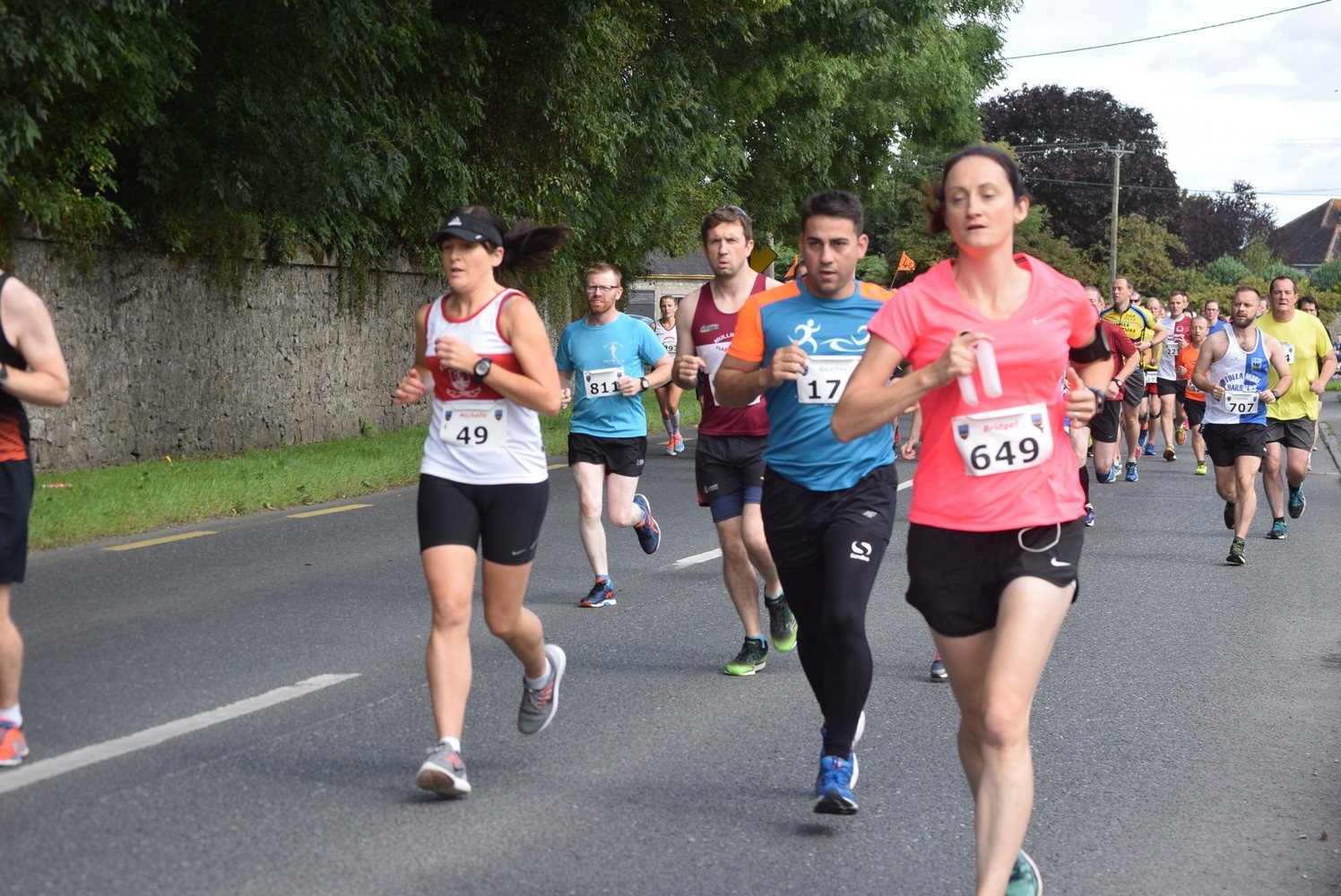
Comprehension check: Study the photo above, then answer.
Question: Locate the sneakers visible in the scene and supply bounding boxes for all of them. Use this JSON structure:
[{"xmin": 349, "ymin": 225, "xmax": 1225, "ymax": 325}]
[
  {"xmin": 724, "ymin": 637, "xmax": 769, "ymax": 676},
  {"xmin": 580, "ymin": 581, "xmax": 617, "ymax": 607},
  {"xmin": 517, "ymin": 643, "xmax": 567, "ymax": 735},
  {"xmin": 820, "ymin": 710, "xmax": 865, "ymax": 750},
  {"xmin": 1085, "ymin": 507, "xmax": 1095, "ymax": 526},
  {"xmin": 1195, "ymin": 462, "xmax": 1207, "ymax": 475},
  {"xmin": 764, "ymin": 582, "xmax": 798, "ymax": 653},
  {"xmin": 1223, "ymin": 501, "xmax": 1235, "ymax": 529},
  {"xmin": 1267, "ymin": 521, "xmax": 1289, "ymax": 539},
  {"xmin": 930, "ymin": 651, "xmax": 948, "ymax": 678},
  {"xmin": 632, "ymin": 494, "xmax": 661, "ymax": 555},
  {"xmin": 415, "ymin": 742, "xmax": 472, "ymax": 798},
  {"xmin": 812, "ymin": 749, "xmax": 860, "ymax": 815},
  {"xmin": 665, "ymin": 438, "xmax": 677, "ymax": 456},
  {"xmin": 674, "ymin": 434, "xmax": 685, "ymax": 452},
  {"xmin": 0, "ymin": 717, "xmax": 30, "ymax": 766},
  {"xmin": 1005, "ymin": 848, "xmax": 1043, "ymax": 896},
  {"xmin": 1225, "ymin": 539, "xmax": 1246, "ymax": 564},
  {"xmin": 1110, "ymin": 463, "xmax": 1122, "ymax": 481},
  {"xmin": 1125, "ymin": 462, "xmax": 1138, "ymax": 482},
  {"xmin": 1117, "ymin": 418, "xmax": 1190, "ymax": 462},
  {"xmin": 1287, "ymin": 480, "xmax": 1306, "ymax": 519}
]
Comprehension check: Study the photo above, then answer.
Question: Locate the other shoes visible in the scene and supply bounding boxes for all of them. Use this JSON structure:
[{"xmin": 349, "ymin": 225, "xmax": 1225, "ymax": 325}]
[{"xmin": 894, "ymin": 430, "xmax": 901, "ymax": 442}]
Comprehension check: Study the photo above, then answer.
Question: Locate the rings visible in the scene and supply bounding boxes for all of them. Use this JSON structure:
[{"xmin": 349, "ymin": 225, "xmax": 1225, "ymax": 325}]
[{"xmin": 1075, "ymin": 412, "xmax": 1079, "ymax": 419}]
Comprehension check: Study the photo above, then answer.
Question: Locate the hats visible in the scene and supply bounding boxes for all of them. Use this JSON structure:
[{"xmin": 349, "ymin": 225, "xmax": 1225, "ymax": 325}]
[{"xmin": 427, "ymin": 212, "xmax": 502, "ymax": 247}]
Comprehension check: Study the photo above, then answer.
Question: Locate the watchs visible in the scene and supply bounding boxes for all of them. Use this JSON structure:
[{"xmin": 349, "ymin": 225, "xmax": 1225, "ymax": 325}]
[
  {"xmin": 639, "ymin": 377, "xmax": 649, "ymax": 392},
  {"xmin": 472, "ymin": 356, "xmax": 492, "ymax": 384},
  {"xmin": 1150, "ymin": 341, "xmax": 1154, "ymax": 348},
  {"xmin": 1088, "ymin": 387, "xmax": 1105, "ymax": 414},
  {"xmin": 1111, "ymin": 377, "xmax": 1123, "ymax": 388},
  {"xmin": 1271, "ymin": 390, "xmax": 1279, "ymax": 401}
]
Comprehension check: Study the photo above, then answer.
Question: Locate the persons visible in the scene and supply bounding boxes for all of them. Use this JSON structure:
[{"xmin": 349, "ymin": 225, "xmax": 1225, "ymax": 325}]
[
  {"xmin": 555, "ymin": 262, "xmax": 674, "ymax": 609},
  {"xmin": 712, "ymin": 191, "xmax": 1338, "ymax": 812},
  {"xmin": 392, "ymin": 207, "xmax": 575, "ymax": 798},
  {"xmin": 649, "ymin": 296, "xmax": 684, "ymax": 456},
  {"xmin": 829, "ymin": 142, "xmax": 1113, "ymax": 896},
  {"xmin": 669, "ymin": 206, "xmax": 798, "ymax": 676},
  {"xmin": 1, "ymin": 268, "xmax": 70, "ymax": 767}
]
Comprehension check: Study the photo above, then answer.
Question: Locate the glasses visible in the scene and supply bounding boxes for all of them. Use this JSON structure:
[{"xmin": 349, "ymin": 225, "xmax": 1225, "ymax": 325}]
[
  {"xmin": 586, "ymin": 284, "xmax": 620, "ymax": 294},
  {"xmin": 713, "ymin": 206, "xmax": 748, "ymax": 219}
]
